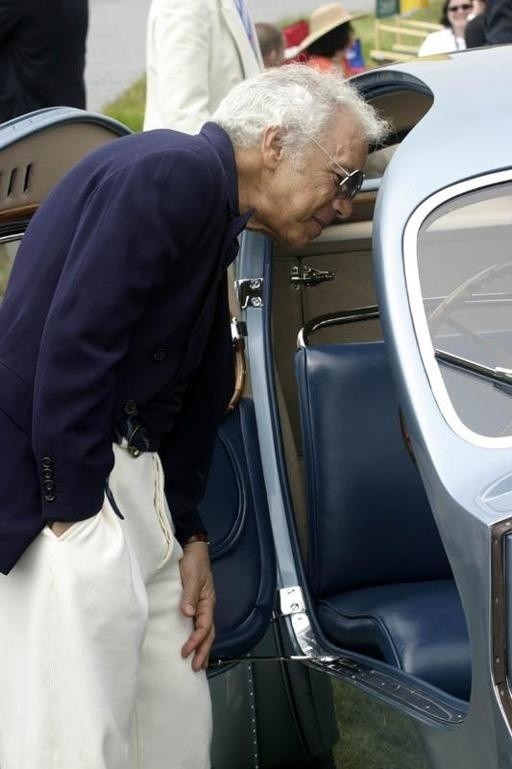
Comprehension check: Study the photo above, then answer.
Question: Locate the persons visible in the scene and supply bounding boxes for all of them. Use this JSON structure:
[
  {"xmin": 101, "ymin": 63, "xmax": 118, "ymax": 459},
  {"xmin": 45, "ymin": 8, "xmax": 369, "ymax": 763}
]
[
  {"xmin": 255, "ymin": 20, "xmax": 284, "ymax": 68},
  {"xmin": 142, "ymin": 0, "xmax": 265, "ymax": 133},
  {"xmin": 296, "ymin": 4, "xmax": 370, "ymax": 79},
  {"xmin": 1, "ymin": 55, "xmax": 390, "ymax": 768},
  {"xmin": 419, "ymin": 0, "xmax": 478, "ymax": 58},
  {"xmin": 346, "ymin": 29, "xmax": 367, "ymax": 74},
  {"xmin": 0, "ymin": 0, "xmax": 88, "ymax": 119},
  {"xmin": 465, "ymin": 0, "xmax": 512, "ymax": 49}
]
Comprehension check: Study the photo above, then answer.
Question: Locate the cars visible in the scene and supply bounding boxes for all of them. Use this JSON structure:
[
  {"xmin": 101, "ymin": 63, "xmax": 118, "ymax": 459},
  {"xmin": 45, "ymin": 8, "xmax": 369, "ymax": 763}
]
[{"xmin": 1, "ymin": 45, "xmax": 512, "ymax": 769}]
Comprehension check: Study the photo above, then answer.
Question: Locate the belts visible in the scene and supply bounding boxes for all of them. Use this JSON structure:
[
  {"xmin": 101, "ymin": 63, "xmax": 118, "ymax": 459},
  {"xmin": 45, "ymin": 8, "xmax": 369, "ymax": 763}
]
[{"xmin": 120, "ymin": 418, "xmax": 161, "ymax": 458}]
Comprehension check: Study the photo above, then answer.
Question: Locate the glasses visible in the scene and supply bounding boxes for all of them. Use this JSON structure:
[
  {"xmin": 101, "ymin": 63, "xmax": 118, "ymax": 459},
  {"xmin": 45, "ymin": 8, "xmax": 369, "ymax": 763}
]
[
  {"xmin": 448, "ymin": 5, "xmax": 472, "ymax": 11},
  {"xmin": 310, "ymin": 137, "xmax": 363, "ymax": 200}
]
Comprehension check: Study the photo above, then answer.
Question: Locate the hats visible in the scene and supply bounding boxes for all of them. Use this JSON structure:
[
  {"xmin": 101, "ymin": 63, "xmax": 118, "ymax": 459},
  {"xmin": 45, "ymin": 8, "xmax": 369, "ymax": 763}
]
[{"xmin": 298, "ymin": 4, "xmax": 369, "ymax": 52}]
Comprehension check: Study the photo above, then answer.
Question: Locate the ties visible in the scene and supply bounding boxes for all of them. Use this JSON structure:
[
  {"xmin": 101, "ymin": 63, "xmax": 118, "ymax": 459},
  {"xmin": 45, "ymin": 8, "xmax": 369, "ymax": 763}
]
[{"xmin": 234, "ymin": 0, "xmax": 252, "ymax": 41}]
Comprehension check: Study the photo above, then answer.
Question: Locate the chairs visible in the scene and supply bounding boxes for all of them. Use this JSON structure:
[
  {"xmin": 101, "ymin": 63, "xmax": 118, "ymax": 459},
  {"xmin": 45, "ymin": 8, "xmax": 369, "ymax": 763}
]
[{"xmin": 289, "ymin": 329, "xmax": 511, "ymax": 698}]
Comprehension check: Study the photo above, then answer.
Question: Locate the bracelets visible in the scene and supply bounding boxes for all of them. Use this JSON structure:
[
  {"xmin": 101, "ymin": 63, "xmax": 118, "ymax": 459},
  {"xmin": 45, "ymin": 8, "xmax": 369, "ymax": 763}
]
[{"xmin": 186, "ymin": 534, "xmax": 209, "ymax": 545}]
